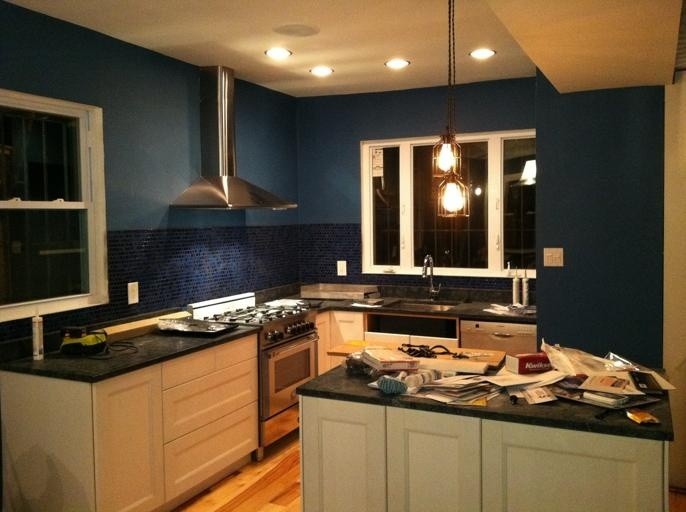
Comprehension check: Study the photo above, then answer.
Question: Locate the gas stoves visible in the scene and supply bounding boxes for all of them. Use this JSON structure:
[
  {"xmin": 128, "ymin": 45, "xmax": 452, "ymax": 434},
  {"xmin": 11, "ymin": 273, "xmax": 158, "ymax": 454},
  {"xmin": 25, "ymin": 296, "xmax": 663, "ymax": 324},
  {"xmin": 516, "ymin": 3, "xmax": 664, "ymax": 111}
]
[{"xmin": 202, "ymin": 302, "xmax": 317, "ymax": 346}]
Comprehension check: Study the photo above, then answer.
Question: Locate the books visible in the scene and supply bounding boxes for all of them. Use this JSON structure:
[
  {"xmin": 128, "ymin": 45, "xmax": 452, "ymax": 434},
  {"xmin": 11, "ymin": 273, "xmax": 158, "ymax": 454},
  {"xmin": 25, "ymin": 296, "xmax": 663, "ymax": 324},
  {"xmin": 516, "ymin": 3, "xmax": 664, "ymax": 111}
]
[{"xmin": 363, "ymin": 349, "xmax": 678, "ymax": 410}]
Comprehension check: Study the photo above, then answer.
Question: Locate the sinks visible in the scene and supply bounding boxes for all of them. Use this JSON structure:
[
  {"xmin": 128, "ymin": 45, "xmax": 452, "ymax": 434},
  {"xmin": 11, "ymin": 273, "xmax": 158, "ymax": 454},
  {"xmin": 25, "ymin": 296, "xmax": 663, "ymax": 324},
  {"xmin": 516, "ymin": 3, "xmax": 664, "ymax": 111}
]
[{"xmin": 383, "ymin": 299, "xmax": 461, "ymax": 312}]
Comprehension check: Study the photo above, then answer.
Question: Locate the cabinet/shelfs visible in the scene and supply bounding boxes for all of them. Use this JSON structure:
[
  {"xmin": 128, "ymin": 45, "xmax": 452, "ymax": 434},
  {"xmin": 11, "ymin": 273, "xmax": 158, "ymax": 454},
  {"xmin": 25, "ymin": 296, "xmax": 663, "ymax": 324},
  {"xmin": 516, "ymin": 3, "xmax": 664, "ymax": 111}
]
[
  {"xmin": 302, "ymin": 396, "xmax": 481, "ymax": 512},
  {"xmin": 315, "ymin": 311, "xmax": 366, "ymax": 374},
  {"xmin": 162, "ymin": 333, "xmax": 259, "ymax": 503},
  {"xmin": 92, "ymin": 363, "xmax": 165, "ymax": 512},
  {"xmin": 481, "ymin": 419, "xmax": 664, "ymax": 512},
  {"xmin": 364, "ymin": 332, "xmax": 458, "ymax": 348}
]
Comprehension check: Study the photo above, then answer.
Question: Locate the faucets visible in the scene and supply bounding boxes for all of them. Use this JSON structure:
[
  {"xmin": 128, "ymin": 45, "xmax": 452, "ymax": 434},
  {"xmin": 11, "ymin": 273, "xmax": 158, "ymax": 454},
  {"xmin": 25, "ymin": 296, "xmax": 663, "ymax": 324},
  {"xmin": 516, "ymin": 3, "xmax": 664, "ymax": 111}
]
[{"xmin": 422, "ymin": 255, "xmax": 441, "ymax": 301}]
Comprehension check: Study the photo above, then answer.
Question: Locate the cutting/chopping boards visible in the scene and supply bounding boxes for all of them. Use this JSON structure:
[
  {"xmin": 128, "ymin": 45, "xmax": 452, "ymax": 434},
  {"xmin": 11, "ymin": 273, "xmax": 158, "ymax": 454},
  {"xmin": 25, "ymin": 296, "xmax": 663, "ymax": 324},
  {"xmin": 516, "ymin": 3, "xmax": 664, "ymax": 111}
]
[{"xmin": 327, "ymin": 340, "xmax": 506, "ymax": 370}]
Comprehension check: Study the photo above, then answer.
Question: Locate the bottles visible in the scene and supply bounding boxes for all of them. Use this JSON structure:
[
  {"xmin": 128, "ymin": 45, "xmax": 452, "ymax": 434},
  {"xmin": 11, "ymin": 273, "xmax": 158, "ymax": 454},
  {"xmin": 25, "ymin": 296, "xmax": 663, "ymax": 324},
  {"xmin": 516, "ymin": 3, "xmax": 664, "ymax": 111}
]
[
  {"xmin": 521, "ymin": 269, "xmax": 530, "ymax": 306},
  {"xmin": 31, "ymin": 309, "xmax": 45, "ymax": 361},
  {"xmin": 512, "ymin": 269, "xmax": 521, "ymax": 307}
]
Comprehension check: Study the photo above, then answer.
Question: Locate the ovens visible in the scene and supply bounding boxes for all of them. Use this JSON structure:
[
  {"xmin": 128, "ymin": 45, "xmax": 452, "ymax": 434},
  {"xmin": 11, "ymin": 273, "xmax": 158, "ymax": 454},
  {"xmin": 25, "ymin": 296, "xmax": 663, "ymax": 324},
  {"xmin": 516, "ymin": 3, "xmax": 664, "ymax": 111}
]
[{"xmin": 259, "ymin": 331, "xmax": 319, "ymax": 448}]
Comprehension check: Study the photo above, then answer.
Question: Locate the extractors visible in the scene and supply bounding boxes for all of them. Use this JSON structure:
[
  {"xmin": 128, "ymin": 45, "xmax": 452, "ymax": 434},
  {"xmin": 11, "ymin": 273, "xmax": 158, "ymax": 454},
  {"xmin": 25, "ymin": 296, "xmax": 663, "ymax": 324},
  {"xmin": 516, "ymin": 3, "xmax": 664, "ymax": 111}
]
[{"xmin": 169, "ymin": 65, "xmax": 299, "ymax": 211}]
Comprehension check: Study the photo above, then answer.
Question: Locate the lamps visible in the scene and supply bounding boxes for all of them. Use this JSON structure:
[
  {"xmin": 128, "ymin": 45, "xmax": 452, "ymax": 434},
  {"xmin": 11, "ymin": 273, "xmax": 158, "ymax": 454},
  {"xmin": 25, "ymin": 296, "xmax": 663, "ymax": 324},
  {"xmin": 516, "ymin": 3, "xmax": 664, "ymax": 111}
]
[
  {"xmin": 437, "ymin": 0, "xmax": 471, "ymax": 218},
  {"xmin": 432, "ymin": 0, "xmax": 462, "ymax": 178}
]
